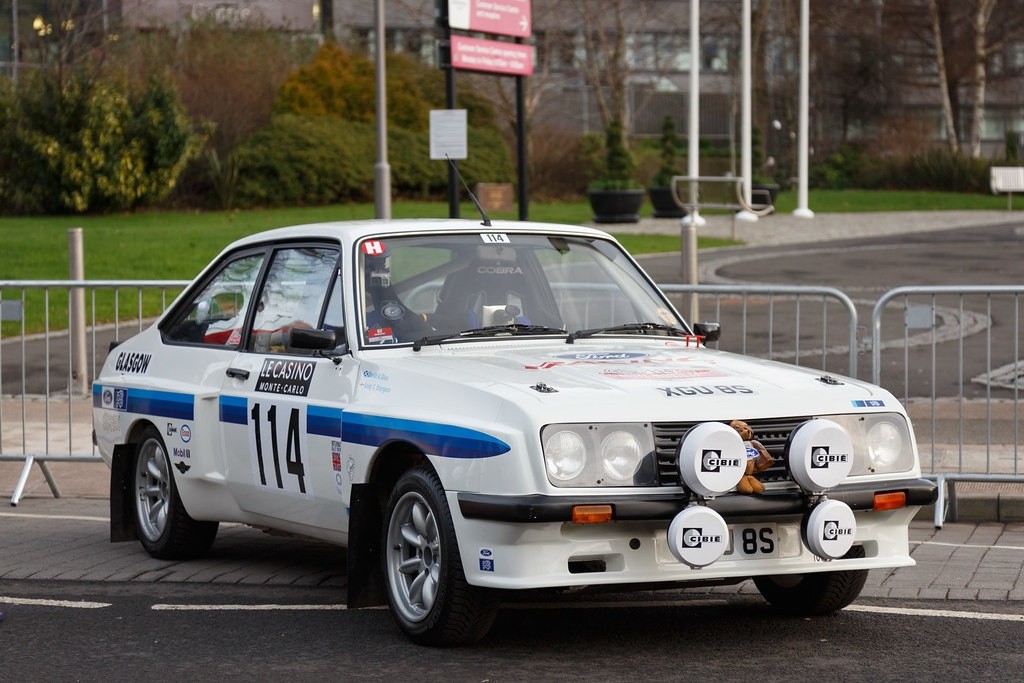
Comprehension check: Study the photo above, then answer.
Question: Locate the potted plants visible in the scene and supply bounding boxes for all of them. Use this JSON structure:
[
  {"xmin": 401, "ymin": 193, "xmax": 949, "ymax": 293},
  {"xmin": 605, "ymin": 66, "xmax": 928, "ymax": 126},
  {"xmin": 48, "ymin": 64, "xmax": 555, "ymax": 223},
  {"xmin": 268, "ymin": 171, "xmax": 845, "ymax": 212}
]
[
  {"xmin": 749, "ymin": 126, "xmax": 777, "ymax": 215},
  {"xmin": 589, "ymin": 114, "xmax": 646, "ymax": 224},
  {"xmin": 649, "ymin": 113, "xmax": 688, "ymax": 217}
]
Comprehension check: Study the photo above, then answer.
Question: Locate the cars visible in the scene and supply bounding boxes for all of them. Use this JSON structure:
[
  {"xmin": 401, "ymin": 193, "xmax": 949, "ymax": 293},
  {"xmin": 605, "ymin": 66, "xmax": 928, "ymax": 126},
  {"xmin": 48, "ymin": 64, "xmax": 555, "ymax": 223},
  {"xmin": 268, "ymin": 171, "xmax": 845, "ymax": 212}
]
[{"xmin": 92, "ymin": 219, "xmax": 940, "ymax": 648}]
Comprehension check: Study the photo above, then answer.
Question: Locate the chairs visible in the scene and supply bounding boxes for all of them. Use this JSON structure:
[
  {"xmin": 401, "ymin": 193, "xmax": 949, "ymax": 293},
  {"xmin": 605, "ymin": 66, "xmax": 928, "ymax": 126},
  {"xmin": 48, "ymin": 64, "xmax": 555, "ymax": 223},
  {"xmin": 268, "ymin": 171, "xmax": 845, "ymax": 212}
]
[{"xmin": 432, "ymin": 259, "xmax": 560, "ymax": 332}]
